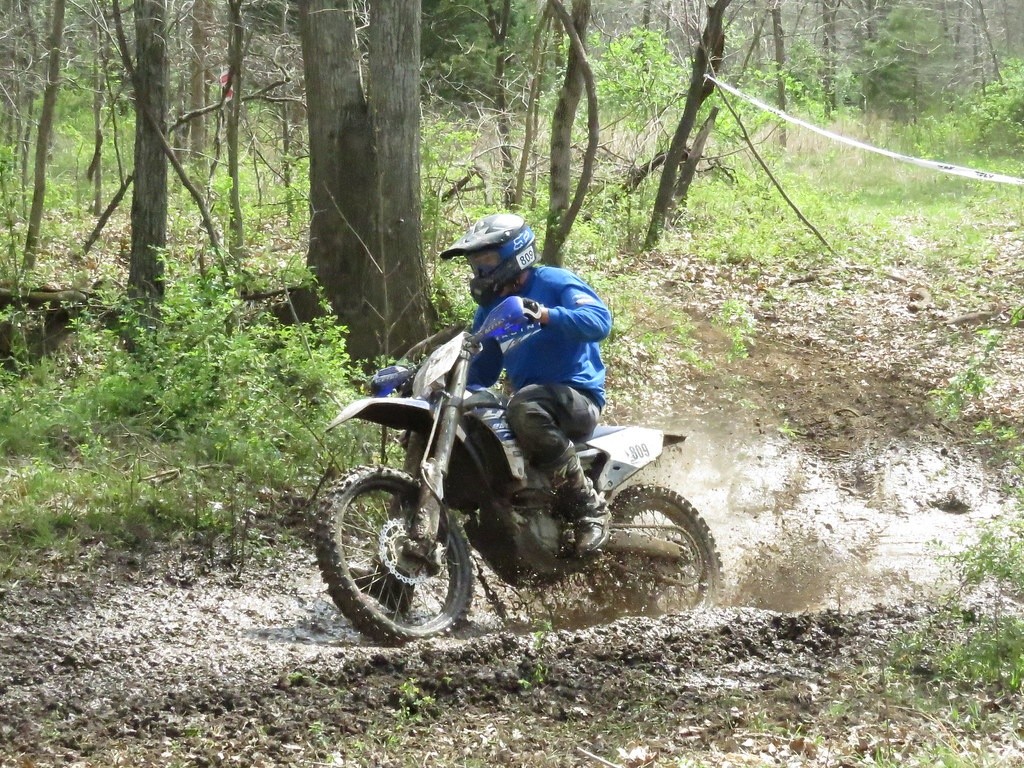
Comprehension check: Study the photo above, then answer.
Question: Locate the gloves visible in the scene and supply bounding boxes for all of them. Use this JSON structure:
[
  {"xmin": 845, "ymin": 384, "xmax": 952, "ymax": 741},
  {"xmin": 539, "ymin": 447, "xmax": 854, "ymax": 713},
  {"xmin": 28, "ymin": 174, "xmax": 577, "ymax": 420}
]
[{"xmin": 522, "ymin": 298, "xmax": 540, "ymax": 324}]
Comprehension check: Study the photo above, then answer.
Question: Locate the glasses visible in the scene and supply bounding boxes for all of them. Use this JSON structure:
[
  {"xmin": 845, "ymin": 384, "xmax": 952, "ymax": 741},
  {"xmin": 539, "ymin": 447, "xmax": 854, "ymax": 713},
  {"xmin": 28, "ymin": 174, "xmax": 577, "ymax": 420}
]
[{"xmin": 461, "ymin": 247, "xmax": 502, "ymax": 277}]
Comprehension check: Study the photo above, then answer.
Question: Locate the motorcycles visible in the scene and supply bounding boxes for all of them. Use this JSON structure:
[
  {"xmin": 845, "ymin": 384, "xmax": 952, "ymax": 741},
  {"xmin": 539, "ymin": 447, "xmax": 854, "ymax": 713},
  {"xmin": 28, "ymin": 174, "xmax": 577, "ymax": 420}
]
[{"xmin": 305, "ymin": 295, "xmax": 727, "ymax": 642}]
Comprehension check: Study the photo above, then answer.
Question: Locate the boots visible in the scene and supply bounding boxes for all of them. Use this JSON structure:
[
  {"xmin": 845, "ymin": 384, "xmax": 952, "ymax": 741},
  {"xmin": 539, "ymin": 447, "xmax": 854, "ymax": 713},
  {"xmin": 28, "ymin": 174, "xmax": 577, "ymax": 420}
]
[{"xmin": 539, "ymin": 439, "xmax": 613, "ymax": 555}]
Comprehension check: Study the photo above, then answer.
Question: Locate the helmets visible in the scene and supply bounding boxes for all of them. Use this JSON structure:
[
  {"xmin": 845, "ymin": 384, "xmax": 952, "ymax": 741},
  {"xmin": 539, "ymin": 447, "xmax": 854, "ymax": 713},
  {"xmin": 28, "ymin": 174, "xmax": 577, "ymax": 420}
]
[{"xmin": 440, "ymin": 212, "xmax": 536, "ymax": 292}]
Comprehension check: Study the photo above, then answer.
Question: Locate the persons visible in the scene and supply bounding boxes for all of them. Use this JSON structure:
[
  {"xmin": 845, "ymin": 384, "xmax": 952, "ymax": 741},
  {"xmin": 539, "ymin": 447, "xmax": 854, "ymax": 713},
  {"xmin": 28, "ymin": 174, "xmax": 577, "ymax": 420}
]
[{"xmin": 441, "ymin": 213, "xmax": 612, "ymax": 553}]
